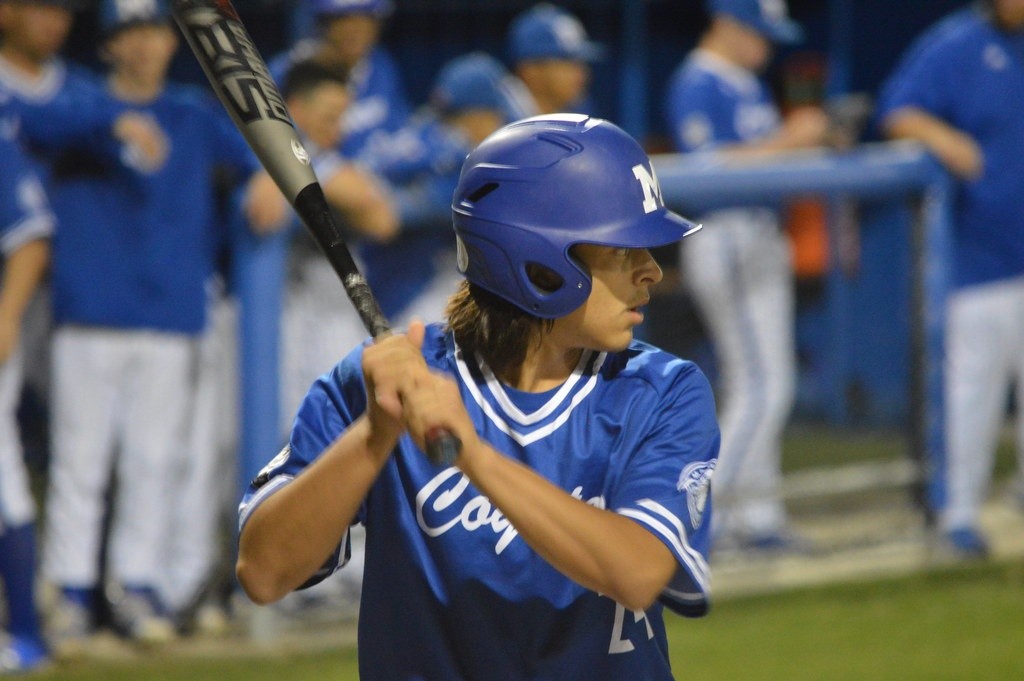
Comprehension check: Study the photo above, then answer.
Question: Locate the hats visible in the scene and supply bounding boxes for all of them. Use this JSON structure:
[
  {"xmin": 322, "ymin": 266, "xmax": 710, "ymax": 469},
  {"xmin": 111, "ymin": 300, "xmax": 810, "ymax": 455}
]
[
  {"xmin": 506, "ymin": 5, "xmax": 611, "ymax": 64},
  {"xmin": 429, "ymin": 49, "xmax": 541, "ymax": 123},
  {"xmin": 96, "ymin": 1, "xmax": 173, "ymax": 39},
  {"xmin": 707, "ymin": 0, "xmax": 807, "ymax": 45}
]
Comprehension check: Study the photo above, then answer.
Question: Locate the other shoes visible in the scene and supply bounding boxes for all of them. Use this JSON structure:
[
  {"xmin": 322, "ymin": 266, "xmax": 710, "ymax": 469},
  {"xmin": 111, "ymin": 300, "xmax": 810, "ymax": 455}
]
[
  {"xmin": 742, "ymin": 537, "xmax": 790, "ymax": 552},
  {"xmin": 45, "ymin": 585, "xmax": 91, "ymax": 663},
  {"xmin": 941, "ymin": 529, "xmax": 990, "ymax": 560},
  {"xmin": 109, "ymin": 581, "xmax": 175, "ymax": 646}
]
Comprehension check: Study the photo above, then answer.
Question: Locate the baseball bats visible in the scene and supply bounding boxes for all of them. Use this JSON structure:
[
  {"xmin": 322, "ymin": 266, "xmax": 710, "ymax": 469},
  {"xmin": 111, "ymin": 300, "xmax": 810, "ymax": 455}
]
[{"xmin": 170, "ymin": 1, "xmax": 463, "ymax": 468}]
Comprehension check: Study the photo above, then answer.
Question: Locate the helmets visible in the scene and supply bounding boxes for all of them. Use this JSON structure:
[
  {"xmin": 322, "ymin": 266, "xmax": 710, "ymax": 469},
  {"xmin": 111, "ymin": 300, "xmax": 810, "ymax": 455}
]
[{"xmin": 450, "ymin": 113, "xmax": 703, "ymax": 319}]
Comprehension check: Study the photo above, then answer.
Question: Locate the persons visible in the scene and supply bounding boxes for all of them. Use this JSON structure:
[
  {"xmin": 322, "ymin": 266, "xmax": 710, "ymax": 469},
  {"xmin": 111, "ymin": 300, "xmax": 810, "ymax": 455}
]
[
  {"xmin": 0, "ymin": 2, "xmax": 1023, "ymax": 680},
  {"xmin": 236, "ymin": 115, "xmax": 719, "ymax": 680},
  {"xmin": 668, "ymin": 1, "xmax": 831, "ymax": 550}
]
